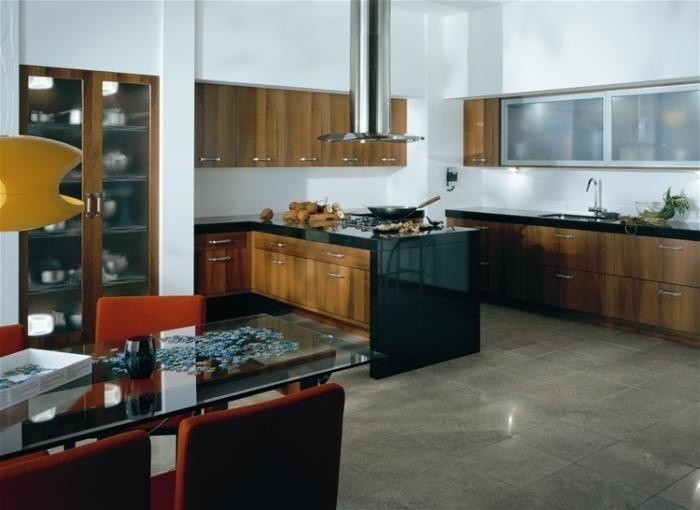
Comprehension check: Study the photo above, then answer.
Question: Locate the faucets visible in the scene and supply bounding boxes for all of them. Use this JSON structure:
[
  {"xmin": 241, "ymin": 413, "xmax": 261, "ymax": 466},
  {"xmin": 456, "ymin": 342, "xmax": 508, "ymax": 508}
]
[{"xmin": 585, "ymin": 178, "xmax": 598, "ymax": 215}]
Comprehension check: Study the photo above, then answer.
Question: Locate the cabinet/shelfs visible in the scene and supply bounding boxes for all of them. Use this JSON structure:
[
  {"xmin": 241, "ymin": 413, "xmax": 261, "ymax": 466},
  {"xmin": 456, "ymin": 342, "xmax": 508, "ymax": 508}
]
[
  {"xmin": 462, "ymin": 98, "xmax": 502, "ymax": 168},
  {"xmin": 194, "ymin": 220, "xmax": 250, "ymax": 300},
  {"xmin": 195, "ymin": 78, "xmax": 287, "ymax": 168},
  {"xmin": 614, "ymin": 223, "xmax": 699, "ymax": 337},
  {"xmin": 286, "ymin": 86, "xmax": 364, "ymax": 168},
  {"xmin": 522, "ymin": 211, "xmax": 614, "ymax": 329},
  {"xmin": 250, "ymin": 219, "xmax": 307, "ymax": 311},
  {"xmin": 444, "ymin": 209, "xmax": 522, "ymax": 311},
  {"xmin": 18, "ymin": 64, "xmax": 162, "ymax": 350},
  {"xmin": 364, "ymin": 98, "xmax": 408, "ymax": 167},
  {"xmin": 306, "ymin": 229, "xmax": 379, "ymax": 333}
]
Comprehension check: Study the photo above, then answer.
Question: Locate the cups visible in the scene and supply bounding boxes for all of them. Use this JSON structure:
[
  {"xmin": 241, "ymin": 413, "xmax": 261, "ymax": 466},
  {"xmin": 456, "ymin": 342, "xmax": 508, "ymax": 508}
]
[{"xmin": 124, "ymin": 335, "xmax": 157, "ymax": 379}]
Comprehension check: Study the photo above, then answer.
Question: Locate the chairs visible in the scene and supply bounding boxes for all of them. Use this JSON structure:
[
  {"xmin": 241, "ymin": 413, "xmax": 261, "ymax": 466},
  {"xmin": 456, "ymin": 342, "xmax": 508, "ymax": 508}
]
[
  {"xmin": 0, "ymin": 323, "xmax": 25, "ymax": 359},
  {"xmin": 94, "ymin": 293, "xmax": 206, "ymax": 444},
  {"xmin": 0, "ymin": 428, "xmax": 152, "ymax": 510},
  {"xmin": 149, "ymin": 381, "xmax": 346, "ymax": 510}
]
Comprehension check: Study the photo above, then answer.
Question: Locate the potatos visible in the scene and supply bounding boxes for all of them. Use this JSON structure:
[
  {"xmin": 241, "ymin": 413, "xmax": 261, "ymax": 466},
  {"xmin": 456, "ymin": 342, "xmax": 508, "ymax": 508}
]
[
  {"xmin": 281, "ymin": 199, "xmax": 344, "ymax": 227},
  {"xmin": 258, "ymin": 208, "xmax": 275, "ymax": 220}
]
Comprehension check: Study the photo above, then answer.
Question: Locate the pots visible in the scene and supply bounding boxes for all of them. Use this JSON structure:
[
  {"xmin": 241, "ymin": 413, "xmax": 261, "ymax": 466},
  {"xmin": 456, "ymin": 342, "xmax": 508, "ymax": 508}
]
[
  {"xmin": 367, "ymin": 194, "xmax": 441, "ymax": 218},
  {"xmin": 30, "ymin": 107, "xmax": 76, "ymax": 123},
  {"xmin": 102, "ymin": 198, "xmax": 119, "ymax": 222},
  {"xmin": 103, "ymin": 106, "xmax": 149, "ymax": 126},
  {"xmin": 33, "ymin": 261, "xmax": 68, "ymax": 284},
  {"xmin": 103, "ymin": 150, "xmax": 133, "ymax": 173}
]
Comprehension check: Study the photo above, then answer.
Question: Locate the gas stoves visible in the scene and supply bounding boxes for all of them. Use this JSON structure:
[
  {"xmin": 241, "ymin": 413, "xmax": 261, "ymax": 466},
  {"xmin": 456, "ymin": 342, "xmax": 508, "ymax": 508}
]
[{"xmin": 326, "ymin": 214, "xmax": 444, "ymax": 232}]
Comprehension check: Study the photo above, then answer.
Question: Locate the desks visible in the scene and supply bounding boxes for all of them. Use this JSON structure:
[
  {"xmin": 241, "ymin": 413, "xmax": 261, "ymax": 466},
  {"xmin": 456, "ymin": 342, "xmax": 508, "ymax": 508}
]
[{"xmin": 0, "ymin": 312, "xmax": 390, "ymax": 461}]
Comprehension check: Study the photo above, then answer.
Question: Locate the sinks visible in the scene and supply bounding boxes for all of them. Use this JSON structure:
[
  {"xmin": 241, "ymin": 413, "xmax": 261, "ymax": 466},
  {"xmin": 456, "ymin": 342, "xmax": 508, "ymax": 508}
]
[{"xmin": 539, "ymin": 213, "xmax": 611, "ymax": 223}]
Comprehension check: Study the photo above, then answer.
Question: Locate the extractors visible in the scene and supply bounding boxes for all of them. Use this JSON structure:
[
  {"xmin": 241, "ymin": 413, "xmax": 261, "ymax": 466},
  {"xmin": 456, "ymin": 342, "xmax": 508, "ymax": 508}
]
[{"xmin": 317, "ymin": 1, "xmax": 426, "ymax": 144}]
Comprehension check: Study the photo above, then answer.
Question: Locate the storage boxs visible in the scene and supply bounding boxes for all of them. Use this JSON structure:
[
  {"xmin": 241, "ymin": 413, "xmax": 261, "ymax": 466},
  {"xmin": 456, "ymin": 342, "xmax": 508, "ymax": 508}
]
[{"xmin": 0, "ymin": 345, "xmax": 93, "ymax": 411}]
